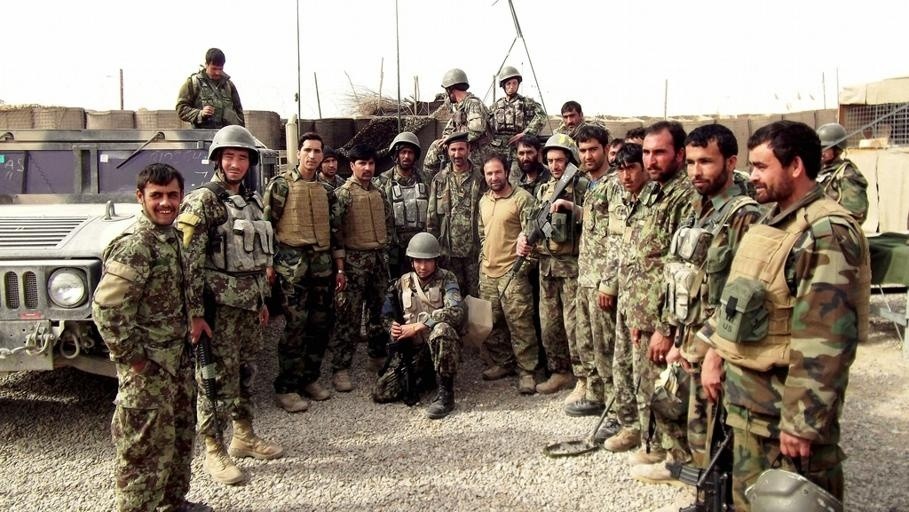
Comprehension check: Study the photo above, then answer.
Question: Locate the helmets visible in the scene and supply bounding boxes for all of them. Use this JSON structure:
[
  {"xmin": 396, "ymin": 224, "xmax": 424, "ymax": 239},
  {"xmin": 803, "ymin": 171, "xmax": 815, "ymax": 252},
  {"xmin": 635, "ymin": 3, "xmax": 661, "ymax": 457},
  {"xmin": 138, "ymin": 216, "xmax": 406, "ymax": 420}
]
[
  {"xmin": 441, "ymin": 69, "xmax": 470, "ymax": 90},
  {"xmin": 541, "ymin": 134, "xmax": 580, "ymax": 165},
  {"xmin": 744, "ymin": 467, "xmax": 843, "ymax": 512},
  {"xmin": 817, "ymin": 123, "xmax": 848, "ymax": 152},
  {"xmin": 208, "ymin": 125, "xmax": 258, "ymax": 166},
  {"xmin": 499, "ymin": 67, "xmax": 522, "ymax": 87},
  {"xmin": 388, "ymin": 132, "xmax": 421, "ymax": 159},
  {"xmin": 406, "ymin": 232, "xmax": 440, "ymax": 259}
]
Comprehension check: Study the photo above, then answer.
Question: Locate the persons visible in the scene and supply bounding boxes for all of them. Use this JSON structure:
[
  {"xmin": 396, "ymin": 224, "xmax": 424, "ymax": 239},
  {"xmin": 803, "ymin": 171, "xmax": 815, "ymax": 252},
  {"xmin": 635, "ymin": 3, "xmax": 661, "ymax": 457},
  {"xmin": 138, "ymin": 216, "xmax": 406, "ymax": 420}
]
[
  {"xmin": 604, "ymin": 142, "xmax": 649, "ymax": 452},
  {"xmin": 92, "ymin": 163, "xmax": 199, "ymax": 512},
  {"xmin": 334, "ymin": 143, "xmax": 396, "ymax": 392},
  {"xmin": 263, "ymin": 132, "xmax": 347, "ymax": 412},
  {"xmin": 478, "ymin": 153, "xmax": 538, "ymax": 394},
  {"xmin": 565, "ymin": 126, "xmax": 628, "ymax": 443},
  {"xmin": 489, "ymin": 66, "xmax": 546, "ymax": 152},
  {"xmin": 557, "ymin": 101, "xmax": 584, "ymax": 136},
  {"xmin": 513, "ymin": 137, "xmax": 551, "ymax": 197},
  {"xmin": 816, "ymin": 124, "xmax": 869, "ymax": 229},
  {"xmin": 179, "ymin": 126, "xmax": 284, "ymax": 485},
  {"xmin": 373, "ymin": 232, "xmax": 468, "ymax": 419},
  {"xmin": 710, "ymin": 121, "xmax": 870, "ymax": 512},
  {"xmin": 516, "ymin": 133, "xmax": 591, "ymax": 405},
  {"xmin": 684, "ymin": 124, "xmax": 761, "ymax": 512},
  {"xmin": 374, "ymin": 132, "xmax": 430, "ymax": 271},
  {"xmin": 625, "ymin": 127, "xmax": 651, "ymax": 148},
  {"xmin": 176, "ymin": 48, "xmax": 245, "ymax": 129},
  {"xmin": 427, "ymin": 132, "xmax": 490, "ymax": 299},
  {"xmin": 317, "ymin": 144, "xmax": 346, "ymax": 189},
  {"xmin": 423, "ymin": 68, "xmax": 495, "ymax": 177},
  {"xmin": 628, "ymin": 122, "xmax": 688, "ymax": 484},
  {"xmin": 606, "ymin": 138, "xmax": 628, "ymax": 167}
]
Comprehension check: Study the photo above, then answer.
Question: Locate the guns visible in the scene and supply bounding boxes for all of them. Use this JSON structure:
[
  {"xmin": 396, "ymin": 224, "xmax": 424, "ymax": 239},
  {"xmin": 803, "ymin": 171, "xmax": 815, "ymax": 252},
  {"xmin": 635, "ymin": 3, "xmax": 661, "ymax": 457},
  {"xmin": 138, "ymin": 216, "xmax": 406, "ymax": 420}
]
[
  {"xmin": 187, "ymin": 281, "xmax": 223, "ymax": 446},
  {"xmin": 666, "ymin": 387, "xmax": 735, "ymax": 512},
  {"xmin": 377, "ymin": 255, "xmax": 421, "ymax": 407},
  {"xmin": 499, "ymin": 163, "xmax": 580, "ymax": 305}
]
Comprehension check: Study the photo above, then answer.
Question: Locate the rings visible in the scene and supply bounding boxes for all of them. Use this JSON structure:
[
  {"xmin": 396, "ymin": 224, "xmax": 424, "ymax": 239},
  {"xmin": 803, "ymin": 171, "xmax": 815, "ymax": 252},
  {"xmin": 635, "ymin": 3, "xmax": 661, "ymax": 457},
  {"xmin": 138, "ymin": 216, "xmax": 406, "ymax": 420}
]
[{"xmin": 659, "ymin": 355, "xmax": 664, "ymax": 359}]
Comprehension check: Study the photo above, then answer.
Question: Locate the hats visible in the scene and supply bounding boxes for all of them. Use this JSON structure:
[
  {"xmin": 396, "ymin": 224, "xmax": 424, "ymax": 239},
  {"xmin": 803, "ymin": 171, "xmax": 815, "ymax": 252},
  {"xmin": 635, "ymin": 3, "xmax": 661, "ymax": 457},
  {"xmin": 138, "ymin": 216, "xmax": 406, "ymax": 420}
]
[
  {"xmin": 321, "ymin": 145, "xmax": 339, "ymax": 163},
  {"xmin": 445, "ymin": 133, "xmax": 468, "ymax": 144}
]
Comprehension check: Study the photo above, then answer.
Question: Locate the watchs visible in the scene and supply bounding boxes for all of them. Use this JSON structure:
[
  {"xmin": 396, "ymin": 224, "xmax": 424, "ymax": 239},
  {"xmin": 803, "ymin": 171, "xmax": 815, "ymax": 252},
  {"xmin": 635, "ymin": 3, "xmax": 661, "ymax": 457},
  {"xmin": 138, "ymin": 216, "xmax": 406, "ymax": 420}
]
[
  {"xmin": 412, "ymin": 323, "xmax": 418, "ymax": 333},
  {"xmin": 338, "ymin": 269, "xmax": 345, "ymax": 276}
]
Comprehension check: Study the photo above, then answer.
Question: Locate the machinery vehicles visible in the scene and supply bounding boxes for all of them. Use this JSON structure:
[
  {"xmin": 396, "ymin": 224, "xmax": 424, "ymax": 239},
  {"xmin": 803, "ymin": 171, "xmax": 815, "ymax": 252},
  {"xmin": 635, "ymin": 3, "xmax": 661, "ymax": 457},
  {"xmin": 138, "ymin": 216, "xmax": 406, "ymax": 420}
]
[{"xmin": 3, "ymin": 114, "xmax": 299, "ymax": 380}]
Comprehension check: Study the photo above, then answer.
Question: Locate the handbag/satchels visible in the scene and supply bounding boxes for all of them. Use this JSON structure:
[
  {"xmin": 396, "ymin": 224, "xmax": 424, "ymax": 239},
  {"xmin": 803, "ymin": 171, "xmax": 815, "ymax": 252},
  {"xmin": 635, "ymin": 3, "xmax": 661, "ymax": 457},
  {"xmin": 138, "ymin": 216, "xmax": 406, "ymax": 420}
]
[{"xmin": 412, "ymin": 272, "xmax": 492, "ymax": 344}]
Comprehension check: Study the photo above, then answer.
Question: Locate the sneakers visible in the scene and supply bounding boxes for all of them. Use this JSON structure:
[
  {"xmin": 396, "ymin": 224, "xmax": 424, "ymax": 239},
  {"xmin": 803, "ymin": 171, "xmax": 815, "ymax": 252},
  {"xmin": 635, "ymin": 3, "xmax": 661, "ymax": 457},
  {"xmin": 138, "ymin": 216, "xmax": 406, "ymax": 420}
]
[
  {"xmin": 536, "ymin": 370, "xmax": 576, "ymax": 394},
  {"xmin": 276, "ymin": 392, "xmax": 307, "ymax": 413},
  {"xmin": 604, "ymin": 425, "xmax": 639, "ymax": 451},
  {"xmin": 186, "ymin": 503, "xmax": 211, "ymax": 512},
  {"xmin": 566, "ymin": 399, "xmax": 607, "ymax": 416},
  {"xmin": 305, "ymin": 381, "xmax": 329, "ymax": 401},
  {"xmin": 630, "ymin": 443, "xmax": 666, "ymax": 464},
  {"xmin": 205, "ymin": 435, "xmax": 244, "ymax": 485},
  {"xmin": 484, "ymin": 366, "xmax": 514, "ymax": 379},
  {"xmin": 564, "ymin": 378, "xmax": 587, "ymax": 404},
  {"xmin": 333, "ymin": 370, "xmax": 354, "ymax": 391},
  {"xmin": 428, "ymin": 382, "xmax": 455, "ymax": 419},
  {"xmin": 519, "ymin": 369, "xmax": 536, "ymax": 394},
  {"xmin": 597, "ymin": 418, "xmax": 621, "ymax": 443},
  {"xmin": 632, "ymin": 451, "xmax": 694, "ymax": 480},
  {"xmin": 227, "ymin": 420, "xmax": 283, "ymax": 460},
  {"xmin": 377, "ymin": 358, "xmax": 386, "ymax": 371}
]
